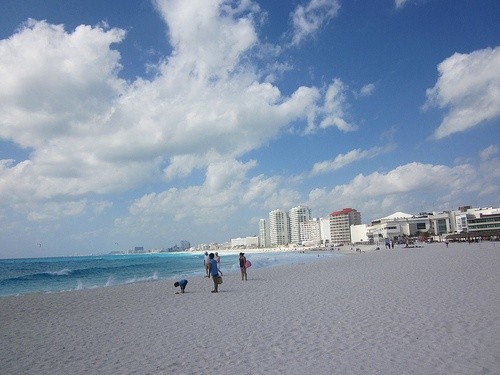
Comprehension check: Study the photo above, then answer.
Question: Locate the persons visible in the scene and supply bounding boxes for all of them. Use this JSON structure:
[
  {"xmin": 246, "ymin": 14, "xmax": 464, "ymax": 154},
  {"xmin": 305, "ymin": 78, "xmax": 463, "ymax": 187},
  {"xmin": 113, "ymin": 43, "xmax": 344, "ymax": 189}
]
[
  {"xmin": 384, "ymin": 236, "xmax": 470, "ymax": 249},
  {"xmin": 238, "ymin": 252, "xmax": 248, "ymax": 280},
  {"xmin": 203, "ymin": 252, "xmax": 222, "ymax": 293},
  {"xmin": 174, "ymin": 279, "xmax": 188, "ymax": 293}
]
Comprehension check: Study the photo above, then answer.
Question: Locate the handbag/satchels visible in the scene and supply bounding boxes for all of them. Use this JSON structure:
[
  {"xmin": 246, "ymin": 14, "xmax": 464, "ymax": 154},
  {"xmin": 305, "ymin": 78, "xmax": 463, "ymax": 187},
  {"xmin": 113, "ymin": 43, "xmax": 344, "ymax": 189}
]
[
  {"xmin": 218, "ymin": 275, "xmax": 222, "ymax": 284},
  {"xmin": 245, "ymin": 260, "xmax": 251, "ymax": 268}
]
